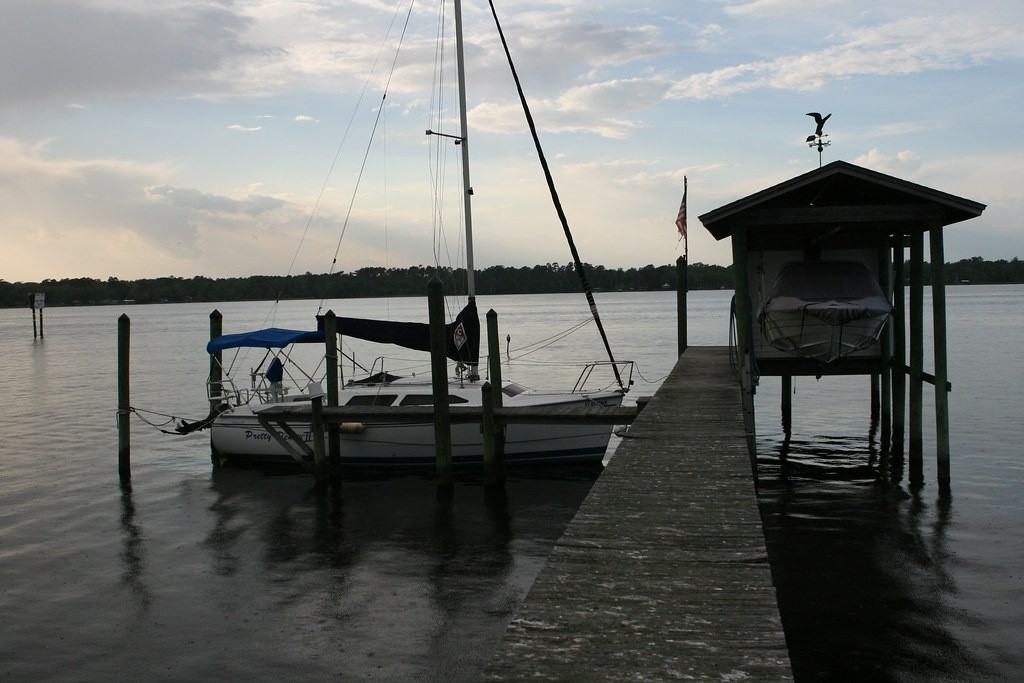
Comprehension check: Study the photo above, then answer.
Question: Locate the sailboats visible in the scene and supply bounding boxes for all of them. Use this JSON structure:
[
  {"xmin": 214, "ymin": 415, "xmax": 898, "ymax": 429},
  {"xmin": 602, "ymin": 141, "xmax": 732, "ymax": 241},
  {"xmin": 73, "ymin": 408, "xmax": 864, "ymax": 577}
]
[{"xmin": 211, "ymin": 0, "xmax": 637, "ymax": 470}]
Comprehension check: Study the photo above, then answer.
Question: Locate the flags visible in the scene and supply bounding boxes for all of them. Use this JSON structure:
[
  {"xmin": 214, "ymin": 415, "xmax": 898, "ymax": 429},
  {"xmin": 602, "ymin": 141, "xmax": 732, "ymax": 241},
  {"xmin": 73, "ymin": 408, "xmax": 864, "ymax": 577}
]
[{"xmin": 675, "ymin": 178, "xmax": 688, "ymax": 241}]
